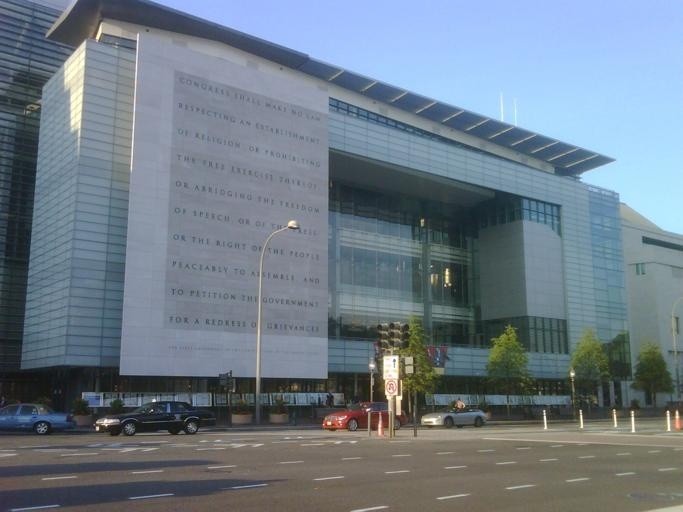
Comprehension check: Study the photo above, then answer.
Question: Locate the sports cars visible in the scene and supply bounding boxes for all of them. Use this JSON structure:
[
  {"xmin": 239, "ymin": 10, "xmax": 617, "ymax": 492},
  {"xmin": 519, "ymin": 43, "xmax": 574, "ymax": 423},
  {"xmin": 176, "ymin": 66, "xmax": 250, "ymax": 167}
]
[{"xmin": 419, "ymin": 403, "xmax": 488, "ymax": 429}]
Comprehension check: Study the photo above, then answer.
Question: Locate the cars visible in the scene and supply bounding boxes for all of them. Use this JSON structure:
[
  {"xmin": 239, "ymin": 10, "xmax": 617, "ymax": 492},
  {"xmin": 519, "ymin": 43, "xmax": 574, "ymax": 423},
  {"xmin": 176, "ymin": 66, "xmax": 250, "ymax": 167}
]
[
  {"xmin": 92, "ymin": 399, "xmax": 217, "ymax": 438},
  {"xmin": 320, "ymin": 401, "xmax": 409, "ymax": 432},
  {"xmin": 0, "ymin": 403, "xmax": 77, "ymax": 437}
]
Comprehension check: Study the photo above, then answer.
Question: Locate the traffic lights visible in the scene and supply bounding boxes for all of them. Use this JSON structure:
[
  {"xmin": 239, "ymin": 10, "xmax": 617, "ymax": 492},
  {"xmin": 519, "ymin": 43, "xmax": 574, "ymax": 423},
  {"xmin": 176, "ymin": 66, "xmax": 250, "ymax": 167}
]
[
  {"xmin": 374, "ymin": 322, "xmax": 388, "ymax": 351},
  {"xmin": 399, "ymin": 323, "xmax": 410, "ymax": 353},
  {"xmin": 387, "ymin": 321, "xmax": 401, "ymax": 351}
]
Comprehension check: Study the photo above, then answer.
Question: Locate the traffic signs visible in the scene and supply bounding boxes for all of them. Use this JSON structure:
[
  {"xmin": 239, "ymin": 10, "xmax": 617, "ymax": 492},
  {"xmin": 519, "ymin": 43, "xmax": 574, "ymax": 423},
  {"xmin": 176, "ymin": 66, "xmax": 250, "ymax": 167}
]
[{"xmin": 381, "ymin": 355, "xmax": 399, "ymax": 381}]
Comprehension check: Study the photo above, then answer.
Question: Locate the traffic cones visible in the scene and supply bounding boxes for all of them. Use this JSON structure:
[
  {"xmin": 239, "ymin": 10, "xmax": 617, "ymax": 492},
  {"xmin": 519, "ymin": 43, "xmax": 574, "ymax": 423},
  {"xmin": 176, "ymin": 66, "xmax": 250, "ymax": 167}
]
[{"xmin": 671, "ymin": 411, "xmax": 681, "ymax": 431}]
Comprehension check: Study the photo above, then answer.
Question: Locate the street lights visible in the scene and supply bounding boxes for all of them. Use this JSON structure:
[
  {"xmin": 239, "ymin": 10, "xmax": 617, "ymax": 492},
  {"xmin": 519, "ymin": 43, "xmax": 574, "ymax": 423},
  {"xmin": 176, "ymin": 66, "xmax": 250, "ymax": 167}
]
[
  {"xmin": 568, "ymin": 367, "xmax": 578, "ymax": 424},
  {"xmin": 367, "ymin": 357, "xmax": 377, "ymax": 402},
  {"xmin": 253, "ymin": 218, "xmax": 300, "ymax": 425}
]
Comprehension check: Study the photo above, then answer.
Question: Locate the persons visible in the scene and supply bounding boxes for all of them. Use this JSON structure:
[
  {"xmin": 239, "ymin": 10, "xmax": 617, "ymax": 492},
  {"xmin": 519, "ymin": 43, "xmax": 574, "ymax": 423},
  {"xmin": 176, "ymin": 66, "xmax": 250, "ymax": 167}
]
[
  {"xmin": 315, "ymin": 391, "xmax": 335, "ymax": 407},
  {"xmin": 454, "ymin": 398, "xmax": 466, "ymax": 428},
  {"xmin": 432, "ymin": 350, "xmax": 442, "ymax": 368}
]
